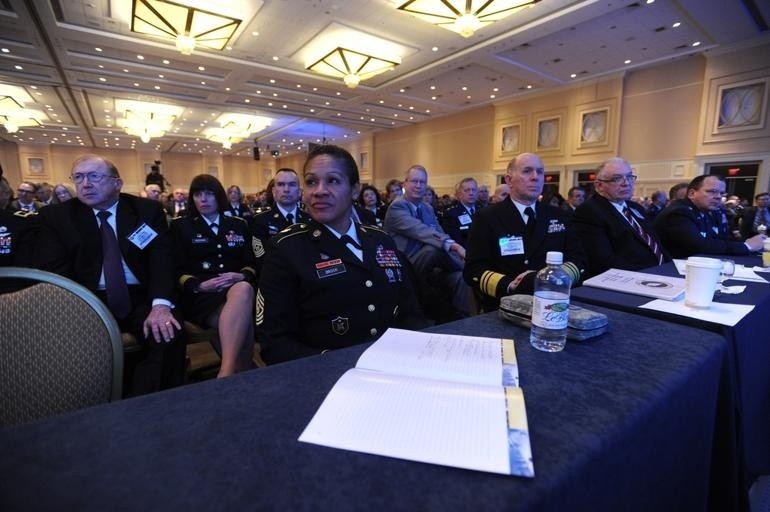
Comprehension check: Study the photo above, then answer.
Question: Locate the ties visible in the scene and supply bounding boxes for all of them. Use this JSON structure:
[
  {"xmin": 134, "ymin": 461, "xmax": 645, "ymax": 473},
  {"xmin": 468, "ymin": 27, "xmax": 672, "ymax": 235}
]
[
  {"xmin": 98, "ymin": 211, "xmax": 134, "ymax": 318},
  {"xmin": 622, "ymin": 207, "xmax": 667, "ymax": 266},
  {"xmin": 703, "ymin": 211, "xmax": 717, "ymax": 227},
  {"xmin": 471, "ymin": 207, "xmax": 476, "ymax": 217},
  {"xmin": 524, "ymin": 206, "xmax": 536, "ymax": 224},
  {"xmin": 286, "ymin": 213, "xmax": 294, "ymax": 225},
  {"xmin": 759, "ymin": 208, "xmax": 767, "ymax": 228},
  {"xmin": 351, "ymin": 210, "xmax": 359, "ymax": 222},
  {"xmin": 417, "ymin": 206, "xmax": 424, "ymax": 221}
]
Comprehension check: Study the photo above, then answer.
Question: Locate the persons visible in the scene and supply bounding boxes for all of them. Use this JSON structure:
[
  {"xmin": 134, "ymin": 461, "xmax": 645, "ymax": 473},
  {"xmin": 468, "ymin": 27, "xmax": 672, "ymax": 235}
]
[{"xmin": 0, "ymin": 140, "xmax": 770, "ymax": 403}]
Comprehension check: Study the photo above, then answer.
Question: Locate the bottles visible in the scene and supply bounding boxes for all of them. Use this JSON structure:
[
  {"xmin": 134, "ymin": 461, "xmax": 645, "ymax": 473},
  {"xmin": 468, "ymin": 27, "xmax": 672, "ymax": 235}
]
[
  {"xmin": 761, "ymin": 237, "xmax": 770, "ymax": 267},
  {"xmin": 529, "ymin": 251, "xmax": 572, "ymax": 353}
]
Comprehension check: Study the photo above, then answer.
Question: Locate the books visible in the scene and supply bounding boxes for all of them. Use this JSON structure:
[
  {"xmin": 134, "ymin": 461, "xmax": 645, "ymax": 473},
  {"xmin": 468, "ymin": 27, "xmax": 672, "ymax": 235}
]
[{"xmin": 297, "ymin": 326, "xmax": 535, "ymax": 483}]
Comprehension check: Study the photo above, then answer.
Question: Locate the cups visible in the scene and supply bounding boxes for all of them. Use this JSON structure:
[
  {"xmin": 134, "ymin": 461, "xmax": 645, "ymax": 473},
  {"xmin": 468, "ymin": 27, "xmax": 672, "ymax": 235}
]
[{"xmin": 684, "ymin": 257, "xmax": 722, "ymax": 309}]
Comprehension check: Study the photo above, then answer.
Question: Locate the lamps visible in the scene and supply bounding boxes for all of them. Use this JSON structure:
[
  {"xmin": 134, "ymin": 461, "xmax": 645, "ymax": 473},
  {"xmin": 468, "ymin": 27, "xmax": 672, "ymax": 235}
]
[
  {"xmin": 0, "ymin": 82, "xmax": 49, "ymax": 137},
  {"xmin": 127, "ymin": 1, "xmax": 247, "ymax": 58},
  {"xmin": 110, "ymin": 97, "xmax": 276, "ymax": 153},
  {"xmin": 398, "ymin": 0, "xmax": 538, "ymax": 41},
  {"xmin": 302, "ymin": 26, "xmax": 404, "ymax": 92}
]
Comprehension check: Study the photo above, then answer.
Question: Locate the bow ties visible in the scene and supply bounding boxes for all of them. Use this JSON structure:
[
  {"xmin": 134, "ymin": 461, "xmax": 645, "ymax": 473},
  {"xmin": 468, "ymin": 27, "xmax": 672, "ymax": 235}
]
[
  {"xmin": 210, "ymin": 223, "xmax": 219, "ymax": 229},
  {"xmin": 233, "ymin": 207, "xmax": 240, "ymax": 210},
  {"xmin": 340, "ymin": 235, "xmax": 362, "ymax": 250}
]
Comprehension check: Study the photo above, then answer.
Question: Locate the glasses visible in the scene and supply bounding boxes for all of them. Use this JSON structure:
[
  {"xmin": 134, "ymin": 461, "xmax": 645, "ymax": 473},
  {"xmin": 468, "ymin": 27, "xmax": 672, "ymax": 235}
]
[
  {"xmin": 596, "ymin": 176, "xmax": 637, "ymax": 185},
  {"xmin": 694, "ymin": 188, "xmax": 728, "ymax": 197},
  {"xmin": 191, "ymin": 191, "xmax": 216, "ymax": 199},
  {"xmin": 757, "ymin": 198, "xmax": 770, "ymax": 202},
  {"xmin": 16, "ymin": 189, "xmax": 37, "ymax": 194},
  {"xmin": 70, "ymin": 172, "xmax": 118, "ymax": 182}
]
